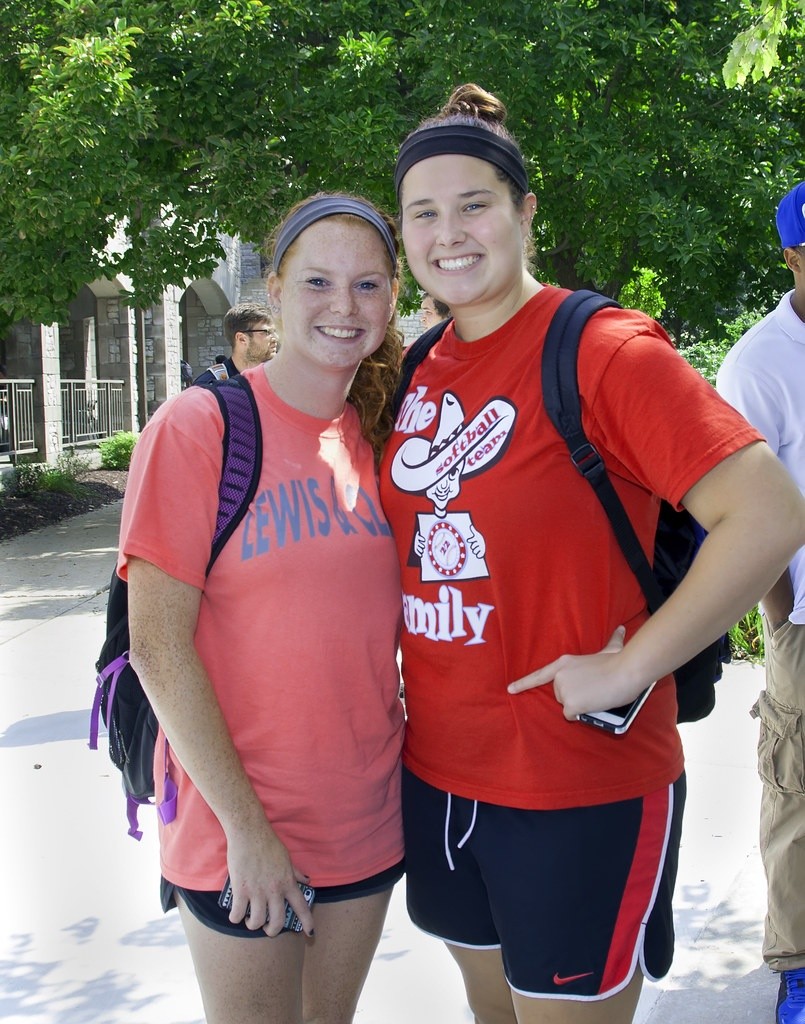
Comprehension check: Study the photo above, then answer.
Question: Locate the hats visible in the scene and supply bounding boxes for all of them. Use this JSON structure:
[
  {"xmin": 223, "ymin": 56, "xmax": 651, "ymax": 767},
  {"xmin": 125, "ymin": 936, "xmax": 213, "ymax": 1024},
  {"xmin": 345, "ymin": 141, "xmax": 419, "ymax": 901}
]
[{"xmin": 776, "ymin": 182, "xmax": 805, "ymax": 248}]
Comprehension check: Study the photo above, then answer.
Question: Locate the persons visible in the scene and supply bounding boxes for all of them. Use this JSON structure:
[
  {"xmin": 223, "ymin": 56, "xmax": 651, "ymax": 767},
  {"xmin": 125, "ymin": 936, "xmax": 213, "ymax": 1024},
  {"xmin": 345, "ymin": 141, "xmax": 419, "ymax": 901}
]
[
  {"xmin": 376, "ymin": 85, "xmax": 805, "ymax": 1024},
  {"xmin": 419, "ymin": 292, "xmax": 450, "ymax": 333},
  {"xmin": 215, "ymin": 355, "xmax": 227, "ymax": 364},
  {"xmin": 191, "ymin": 303, "xmax": 278, "ymax": 386},
  {"xmin": 712, "ymin": 184, "xmax": 805, "ymax": 1024},
  {"xmin": 118, "ymin": 192, "xmax": 414, "ymax": 1024},
  {"xmin": 180, "ymin": 359, "xmax": 186, "ymax": 391}
]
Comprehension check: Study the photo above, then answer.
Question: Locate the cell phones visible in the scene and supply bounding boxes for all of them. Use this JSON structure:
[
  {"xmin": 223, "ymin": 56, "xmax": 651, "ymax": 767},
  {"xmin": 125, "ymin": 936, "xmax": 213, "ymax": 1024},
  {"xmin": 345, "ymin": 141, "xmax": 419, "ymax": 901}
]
[
  {"xmin": 576, "ymin": 680, "xmax": 657, "ymax": 734},
  {"xmin": 218, "ymin": 873, "xmax": 315, "ymax": 933}
]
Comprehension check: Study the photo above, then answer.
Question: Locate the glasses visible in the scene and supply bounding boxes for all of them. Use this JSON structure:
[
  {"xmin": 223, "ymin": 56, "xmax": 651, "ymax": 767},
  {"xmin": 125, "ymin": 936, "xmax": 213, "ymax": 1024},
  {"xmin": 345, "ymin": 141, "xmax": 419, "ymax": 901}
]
[{"xmin": 233, "ymin": 328, "xmax": 278, "ymax": 338}]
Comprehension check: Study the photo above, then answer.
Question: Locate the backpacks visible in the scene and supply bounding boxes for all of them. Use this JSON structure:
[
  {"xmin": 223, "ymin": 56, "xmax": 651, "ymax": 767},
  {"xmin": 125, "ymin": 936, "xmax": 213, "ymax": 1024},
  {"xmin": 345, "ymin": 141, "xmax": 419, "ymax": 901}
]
[
  {"xmin": 90, "ymin": 377, "xmax": 263, "ymax": 840},
  {"xmin": 380, "ymin": 288, "xmax": 729, "ymax": 723}
]
